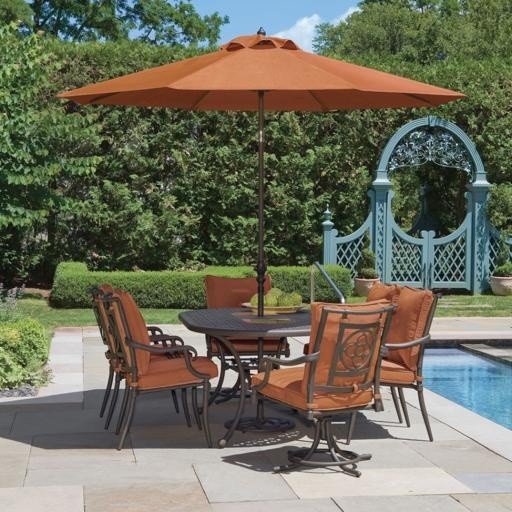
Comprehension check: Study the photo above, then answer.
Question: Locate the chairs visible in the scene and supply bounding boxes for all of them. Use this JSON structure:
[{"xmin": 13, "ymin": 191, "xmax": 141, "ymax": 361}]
[{"xmin": 88, "ymin": 272, "xmax": 437, "ymax": 479}]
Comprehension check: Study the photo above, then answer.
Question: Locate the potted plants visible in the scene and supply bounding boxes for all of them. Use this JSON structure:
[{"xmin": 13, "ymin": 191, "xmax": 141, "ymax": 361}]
[
  {"xmin": 353, "ymin": 247, "xmax": 383, "ymax": 295},
  {"xmin": 489, "ymin": 239, "xmax": 512, "ymax": 297}
]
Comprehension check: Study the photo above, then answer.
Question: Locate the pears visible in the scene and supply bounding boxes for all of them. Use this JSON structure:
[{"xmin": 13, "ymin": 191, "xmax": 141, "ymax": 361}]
[{"xmin": 250, "ymin": 287, "xmax": 302, "ymax": 306}]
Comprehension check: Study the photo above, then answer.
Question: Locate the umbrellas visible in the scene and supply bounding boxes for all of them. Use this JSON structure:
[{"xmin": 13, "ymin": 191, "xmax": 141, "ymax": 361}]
[{"xmin": 53, "ymin": 25, "xmax": 468, "ymax": 419}]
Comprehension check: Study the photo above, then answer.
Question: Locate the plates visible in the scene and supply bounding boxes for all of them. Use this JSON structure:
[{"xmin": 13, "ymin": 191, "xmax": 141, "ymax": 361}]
[{"xmin": 241, "ymin": 302, "xmax": 306, "ymax": 314}]
